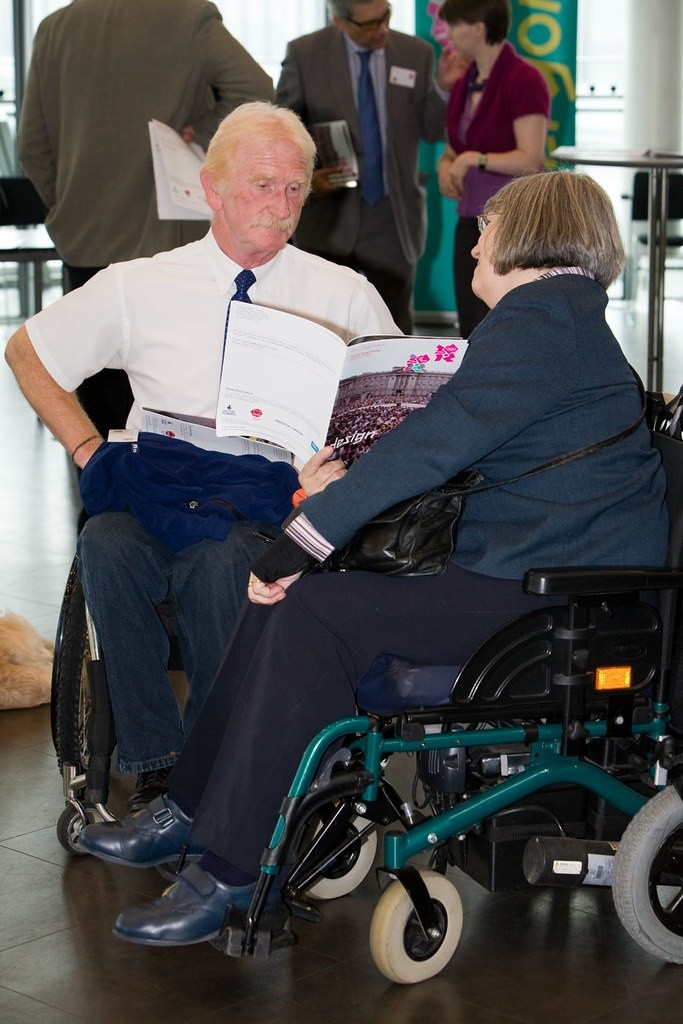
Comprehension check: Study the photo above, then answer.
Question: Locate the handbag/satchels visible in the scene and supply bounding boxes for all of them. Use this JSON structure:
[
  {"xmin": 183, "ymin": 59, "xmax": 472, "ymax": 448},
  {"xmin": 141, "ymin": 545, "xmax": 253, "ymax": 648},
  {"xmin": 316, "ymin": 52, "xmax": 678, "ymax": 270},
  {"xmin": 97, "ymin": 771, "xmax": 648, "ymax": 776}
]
[{"xmin": 320, "ymin": 488, "xmax": 464, "ymax": 577}]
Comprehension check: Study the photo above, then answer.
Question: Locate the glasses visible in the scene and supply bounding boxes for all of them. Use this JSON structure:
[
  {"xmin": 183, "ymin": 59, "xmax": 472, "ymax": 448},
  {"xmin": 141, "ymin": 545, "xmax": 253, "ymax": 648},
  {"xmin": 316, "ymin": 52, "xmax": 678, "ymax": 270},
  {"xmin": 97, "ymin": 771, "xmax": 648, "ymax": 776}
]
[
  {"xmin": 476, "ymin": 212, "xmax": 503, "ymax": 233},
  {"xmin": 347, "ymin": 3, "xmax": 392, "ymax": 32}
]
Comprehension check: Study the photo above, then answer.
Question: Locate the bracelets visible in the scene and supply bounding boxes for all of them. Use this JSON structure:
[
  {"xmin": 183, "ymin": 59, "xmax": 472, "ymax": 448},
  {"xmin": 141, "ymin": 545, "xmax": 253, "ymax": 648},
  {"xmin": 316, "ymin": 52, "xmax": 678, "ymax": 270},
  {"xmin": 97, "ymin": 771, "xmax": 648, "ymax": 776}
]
[
  {"xmin": 72, "ymin": 436, "xmax": 101, "ymax": 463},
  {"xmin": 478, "ymin": 153, "xmax": 485, "ymax": 172}
]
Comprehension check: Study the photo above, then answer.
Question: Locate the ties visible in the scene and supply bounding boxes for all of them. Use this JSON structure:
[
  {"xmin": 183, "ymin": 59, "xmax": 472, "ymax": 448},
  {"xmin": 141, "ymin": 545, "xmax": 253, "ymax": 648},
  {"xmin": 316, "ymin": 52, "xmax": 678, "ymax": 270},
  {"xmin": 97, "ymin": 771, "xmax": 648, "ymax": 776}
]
[
  {"xmin": 218, "ymin": 269, "xmax": 257, "ymax": 385},
  {"xmin": 355, "ymin": 50, "xmax": 383, "ymax": 206}
]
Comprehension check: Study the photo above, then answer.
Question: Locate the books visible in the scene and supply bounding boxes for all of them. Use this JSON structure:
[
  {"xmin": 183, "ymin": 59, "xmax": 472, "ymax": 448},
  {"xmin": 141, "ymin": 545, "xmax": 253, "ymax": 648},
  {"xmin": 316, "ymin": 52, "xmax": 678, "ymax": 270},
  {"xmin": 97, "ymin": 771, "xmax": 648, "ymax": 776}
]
[
  {"xmin": 302, "ymin": 120, "xmax": 358, "ymax": 184},
  {"xmin": 216, "ymin": 301, "xmax": 468, "ymax": 470},
  {"xmin": 141, "ymin": 406, "xmax": 295, "ymax": 466}
]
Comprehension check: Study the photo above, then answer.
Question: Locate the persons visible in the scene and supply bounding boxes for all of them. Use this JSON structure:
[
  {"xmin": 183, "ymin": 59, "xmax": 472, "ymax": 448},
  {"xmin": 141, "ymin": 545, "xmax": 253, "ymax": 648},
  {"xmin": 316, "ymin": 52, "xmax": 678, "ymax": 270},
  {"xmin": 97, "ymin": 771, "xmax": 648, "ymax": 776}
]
[
  {"xmin": 5, "ymin": 101, "xmax": 404, "ymax": 884},
  {"xmin": 18, "ymin": 1, "xmax": 275, "ymax": 297},
  {"xmin": 276, "ymin": 0, "xmax": 471, "ymax": 340},
  {"xmin": 78, "ymin": 172, "xmax": 668, "ymax": 946},
  {"xmin": 440, "ymin": 0, "xmax": 550, "ymax": 341}
]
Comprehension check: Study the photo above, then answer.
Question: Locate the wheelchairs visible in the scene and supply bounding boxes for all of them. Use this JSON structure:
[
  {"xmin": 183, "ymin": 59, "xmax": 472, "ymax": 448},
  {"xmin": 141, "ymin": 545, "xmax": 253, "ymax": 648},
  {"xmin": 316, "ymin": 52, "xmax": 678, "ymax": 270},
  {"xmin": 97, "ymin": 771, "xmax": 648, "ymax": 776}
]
[{"xmin": 47, "ymin": 428, "xmax": 683, "ymax": 986}]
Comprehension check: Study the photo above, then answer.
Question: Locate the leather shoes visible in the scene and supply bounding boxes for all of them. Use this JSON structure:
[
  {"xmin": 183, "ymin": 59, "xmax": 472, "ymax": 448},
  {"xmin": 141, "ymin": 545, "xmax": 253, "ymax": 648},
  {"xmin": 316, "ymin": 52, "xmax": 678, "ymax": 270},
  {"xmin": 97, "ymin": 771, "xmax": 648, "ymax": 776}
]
[
  {"xmin": 77, "ymin": 792, "xmax": 204, "ymax": 869},
  {"xmin": 112, "ymin": 862, "xmax": 285, "ymax": 947},
  {"xmin": 127, "ymin": 768, "xmax": 171, "ymax": 813}
]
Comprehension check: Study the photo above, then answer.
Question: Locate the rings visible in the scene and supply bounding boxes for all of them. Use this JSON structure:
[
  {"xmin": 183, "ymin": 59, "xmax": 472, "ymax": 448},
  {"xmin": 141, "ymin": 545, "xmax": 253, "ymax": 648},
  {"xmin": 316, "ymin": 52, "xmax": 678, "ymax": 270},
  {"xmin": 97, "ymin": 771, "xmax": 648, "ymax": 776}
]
[{"xmin": 249, "ymin": 579, "xmax": 259, "ymax": 586}]
